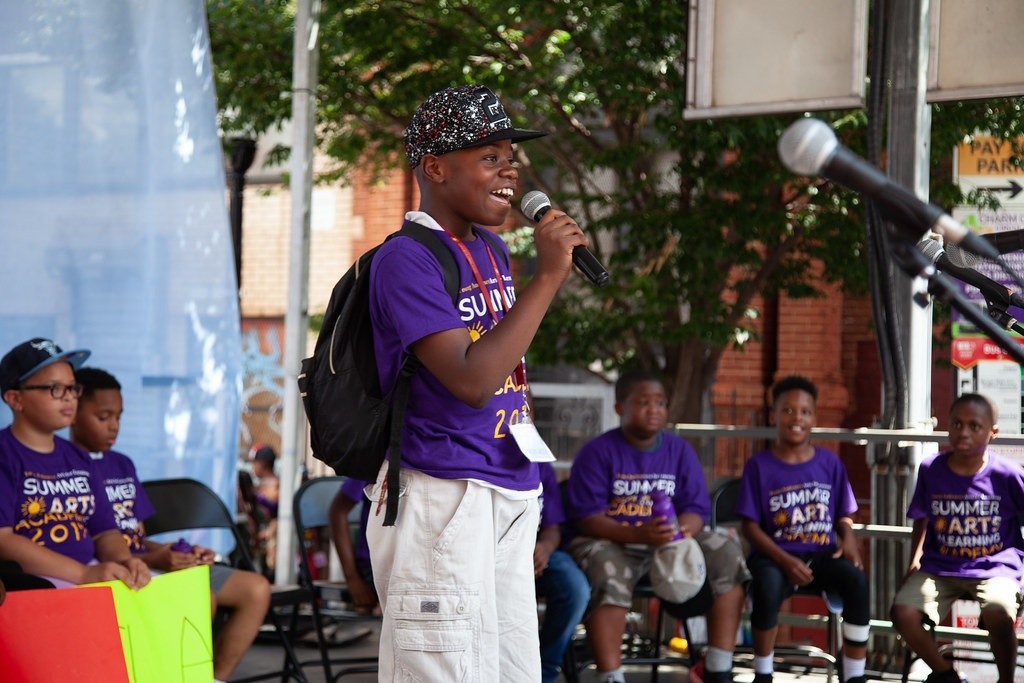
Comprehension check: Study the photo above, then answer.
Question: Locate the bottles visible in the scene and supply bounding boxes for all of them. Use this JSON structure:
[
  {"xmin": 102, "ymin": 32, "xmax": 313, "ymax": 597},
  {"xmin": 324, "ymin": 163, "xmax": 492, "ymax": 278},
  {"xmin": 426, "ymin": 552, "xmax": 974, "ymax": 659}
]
[{"xmin": 649, "ymin": 484, "xmax": 683, "ymax": 540}]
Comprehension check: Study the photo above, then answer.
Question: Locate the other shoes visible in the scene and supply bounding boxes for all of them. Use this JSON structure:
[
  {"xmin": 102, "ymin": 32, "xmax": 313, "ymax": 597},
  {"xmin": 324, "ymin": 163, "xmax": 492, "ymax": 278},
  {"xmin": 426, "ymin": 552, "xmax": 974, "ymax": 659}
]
[
  {"xmin": 836, "ymin": 647, "xmax": 867, "ymax": 683},
  {"xmin": 923, "ymin": 667, "xmax": 961, "ymax": 683},
  {"xmin": 700, "ymin": 652, "xmax": 735, "ymax": 683},
  {"xmin": 750, "ymin": 671, "xmax": 773, "ymax": 683}
]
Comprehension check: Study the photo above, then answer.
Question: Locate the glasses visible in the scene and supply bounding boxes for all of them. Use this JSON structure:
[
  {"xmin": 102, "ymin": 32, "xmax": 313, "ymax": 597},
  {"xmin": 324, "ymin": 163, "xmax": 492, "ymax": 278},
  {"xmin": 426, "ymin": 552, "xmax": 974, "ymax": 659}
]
[{"xmin": 20, "ymin": 383, "xmax": 83, "ymax": 398}]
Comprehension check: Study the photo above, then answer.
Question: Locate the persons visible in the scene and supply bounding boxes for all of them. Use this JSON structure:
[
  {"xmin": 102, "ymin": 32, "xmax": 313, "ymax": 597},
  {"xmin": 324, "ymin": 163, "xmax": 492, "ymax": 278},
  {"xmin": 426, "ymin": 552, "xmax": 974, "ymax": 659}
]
[
  {"xmin": 71, "ymin": 367, "xmax": 271, "ymax": 683},
  {"xmin": 570, "ymin": 367, "xmax": 752, "ymax": 683},
  {"xmin": 891, "ymin": 393, "xmax": 1024, "ymax": 683},
  {"xmin": 235, "ymin": 444, "xmax": 589, "ymax": 683},
  {"xmin": 299, "ymin": 81, "xmax": 589, "ymax": 683},
  {"xmin": 0, "ymin": 337, "xmax": 152, "ymax": 592},
  {"xmin": 737, "ymin": 374, "xmax": 871, "ymax": 683}
]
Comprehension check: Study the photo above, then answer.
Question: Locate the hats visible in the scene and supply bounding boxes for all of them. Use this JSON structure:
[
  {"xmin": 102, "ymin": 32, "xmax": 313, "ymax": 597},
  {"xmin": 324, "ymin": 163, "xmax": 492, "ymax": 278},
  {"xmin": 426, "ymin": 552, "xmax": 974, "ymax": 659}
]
[
  {"xmin": 1, "ymin": 338, "xmax": 91, "ymax": 402},
  {"xmin": 245, "ymin": 442, "xmax": 276, "ymax": 464},
  {"xmin": 401, "ymin": 82, "xmax": 550, "ymax": 170},
  {"xmin": 648, "ymin": 536, "xmax": 714, "ymax": 620}
]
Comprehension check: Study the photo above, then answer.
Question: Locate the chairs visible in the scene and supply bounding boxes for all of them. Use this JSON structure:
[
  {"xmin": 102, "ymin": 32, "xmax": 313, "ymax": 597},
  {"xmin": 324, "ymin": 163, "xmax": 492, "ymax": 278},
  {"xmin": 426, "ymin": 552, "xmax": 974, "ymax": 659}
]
[{"xmin": 133, "ymin": 455, "xmax": 1024, "ymax": 683}]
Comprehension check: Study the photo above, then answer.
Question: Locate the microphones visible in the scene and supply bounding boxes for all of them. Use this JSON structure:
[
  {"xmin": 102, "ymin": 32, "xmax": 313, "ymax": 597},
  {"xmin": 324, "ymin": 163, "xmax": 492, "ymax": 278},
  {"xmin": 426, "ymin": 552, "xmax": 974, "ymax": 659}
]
[
  {"xmin": 778, "ymin": 120, "xmax": 999, "ymax": 261},
  {"xmin": 521, "ymin": 191, "xmax": 609, "ymax": 288},
  {"xmin": 916, "ymin": 239, "xmax": 1024, "ymax": 311}
]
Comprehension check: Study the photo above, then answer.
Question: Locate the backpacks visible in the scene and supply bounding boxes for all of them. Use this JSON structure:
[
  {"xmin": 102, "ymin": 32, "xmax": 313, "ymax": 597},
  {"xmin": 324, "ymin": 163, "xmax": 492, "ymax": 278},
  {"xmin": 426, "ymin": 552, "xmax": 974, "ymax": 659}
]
[{"xmin": 297, "ymin": 219, "xmax": 509, "ymax": 526}]
